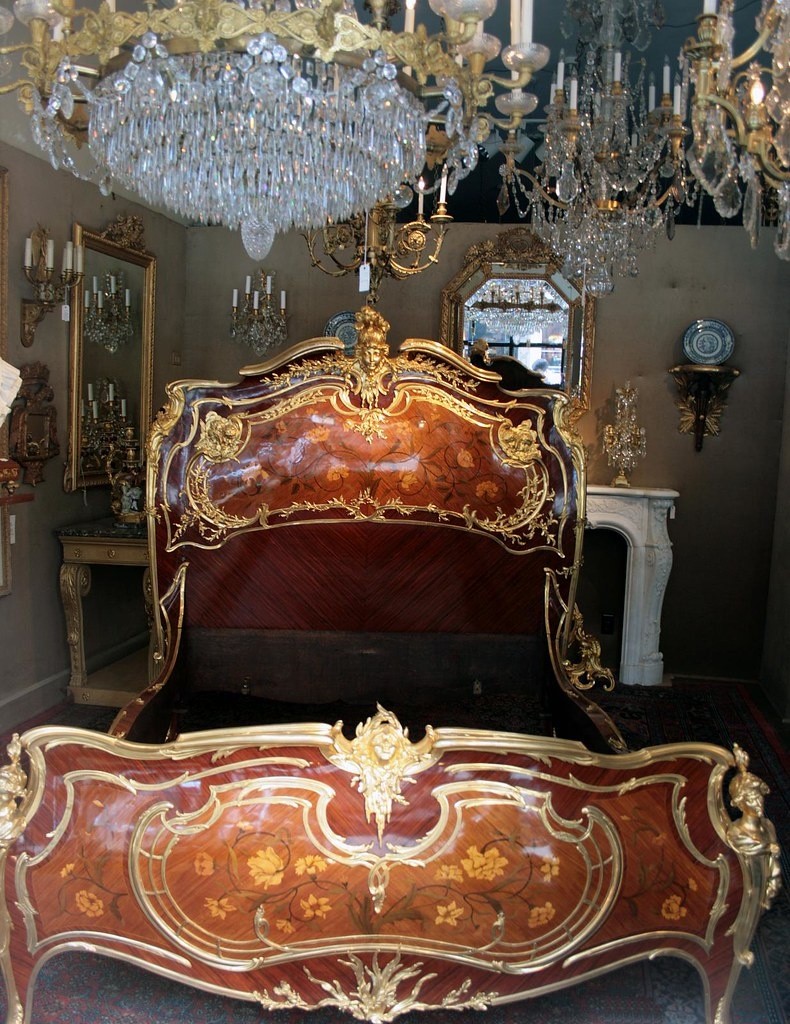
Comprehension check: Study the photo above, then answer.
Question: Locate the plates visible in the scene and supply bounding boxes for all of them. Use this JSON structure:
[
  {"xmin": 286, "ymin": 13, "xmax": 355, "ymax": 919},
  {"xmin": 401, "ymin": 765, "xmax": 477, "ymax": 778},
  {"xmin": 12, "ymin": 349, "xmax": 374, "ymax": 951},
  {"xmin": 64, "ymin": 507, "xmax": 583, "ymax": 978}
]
[
  {"xmin": 324, "ymin": 310, "xmax": 360, "ymax": 356},
  {"xmin": 682, "ymin": 317, "xmax": 735, "ymax": 365}
]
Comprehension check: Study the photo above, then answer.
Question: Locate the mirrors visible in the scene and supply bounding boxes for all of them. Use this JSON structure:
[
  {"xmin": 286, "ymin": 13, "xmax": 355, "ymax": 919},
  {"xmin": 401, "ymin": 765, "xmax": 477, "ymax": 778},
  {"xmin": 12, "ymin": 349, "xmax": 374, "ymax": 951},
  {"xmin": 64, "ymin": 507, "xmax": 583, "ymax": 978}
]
[
  {"xmin": 62, "ymin": 213, "xmax": 156, "ymax": 493},
  {"xmin": 439, "ymin": 226, "xmax": 597, "ymax": 425}
]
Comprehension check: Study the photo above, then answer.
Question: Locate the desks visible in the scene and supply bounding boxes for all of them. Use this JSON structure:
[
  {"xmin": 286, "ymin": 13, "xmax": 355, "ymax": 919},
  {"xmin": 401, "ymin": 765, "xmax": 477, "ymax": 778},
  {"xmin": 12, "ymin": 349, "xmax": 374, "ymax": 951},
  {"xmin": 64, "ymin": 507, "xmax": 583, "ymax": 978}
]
[
  {"xmin": 583, "ymin": 484, "xmax": 679, "ymax": 684},
  {"xmin": 56, "ymin": 517, "xmax": 169, "ymax": 708}
]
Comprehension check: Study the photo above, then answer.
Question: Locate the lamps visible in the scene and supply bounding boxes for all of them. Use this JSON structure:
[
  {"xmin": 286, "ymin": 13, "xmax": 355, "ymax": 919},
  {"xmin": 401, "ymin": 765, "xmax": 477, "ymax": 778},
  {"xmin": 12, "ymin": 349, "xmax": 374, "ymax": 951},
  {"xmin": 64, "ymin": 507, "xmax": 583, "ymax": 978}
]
[{"xmin": 1, "ymin": 0, "xmax": 790, "ymax": 493}]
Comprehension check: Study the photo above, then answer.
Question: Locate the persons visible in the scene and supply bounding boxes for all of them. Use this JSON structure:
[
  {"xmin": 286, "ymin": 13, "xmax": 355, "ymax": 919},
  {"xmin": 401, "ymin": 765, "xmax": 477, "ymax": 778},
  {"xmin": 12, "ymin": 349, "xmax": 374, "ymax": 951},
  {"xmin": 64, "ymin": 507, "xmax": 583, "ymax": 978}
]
[
  {"xmin": 727, "ymin": 745, "xmax": 780, "ymax": 855},
  {"xmin": 353, "ymin": 306, "xmax": 390, "ymax": 376}
]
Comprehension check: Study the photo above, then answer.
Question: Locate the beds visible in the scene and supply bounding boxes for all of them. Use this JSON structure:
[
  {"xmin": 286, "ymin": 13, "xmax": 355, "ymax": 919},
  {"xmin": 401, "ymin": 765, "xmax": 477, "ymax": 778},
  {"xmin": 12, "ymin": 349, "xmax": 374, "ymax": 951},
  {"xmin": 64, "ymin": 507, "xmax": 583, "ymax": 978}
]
[{"xmin": 0, "ymin": 307, "xmax": 782, "ymax": 1024}]
